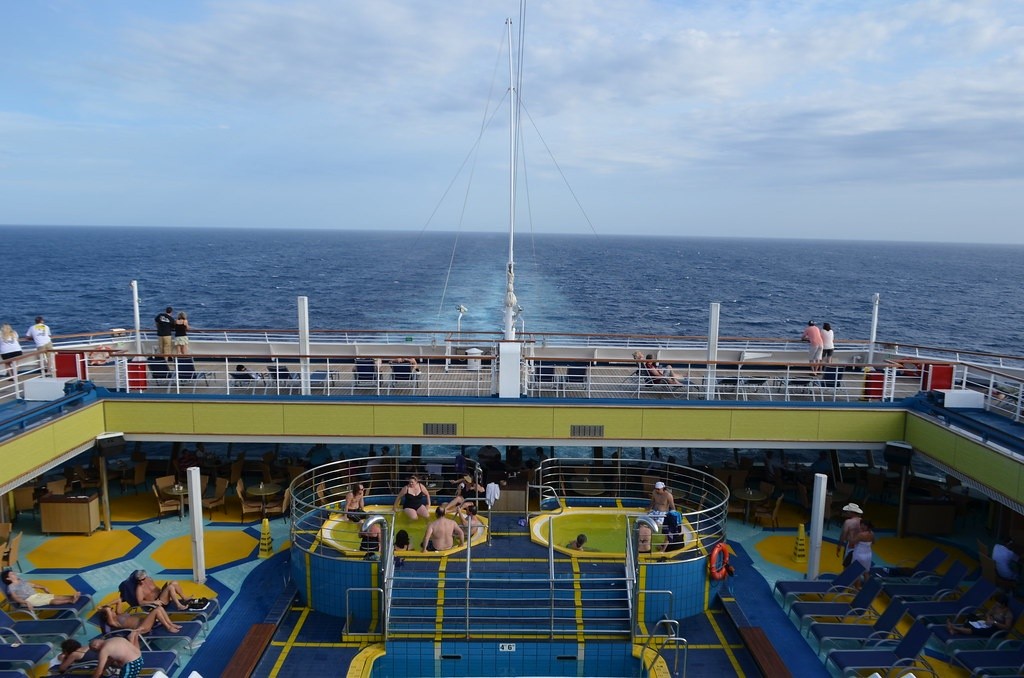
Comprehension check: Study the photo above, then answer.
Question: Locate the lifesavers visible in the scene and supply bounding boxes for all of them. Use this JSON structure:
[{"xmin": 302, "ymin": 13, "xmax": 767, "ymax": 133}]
[
  {"xmin": 899, "ymin": 359, "xmax": 922, "ymax": 376},
  {"xmin": 89, "ymin": 346, "xmax": 111, "ymax": 365},
  {"xmin": 710, "ymin": 543, "xmax": 729, "ymax": 580}
]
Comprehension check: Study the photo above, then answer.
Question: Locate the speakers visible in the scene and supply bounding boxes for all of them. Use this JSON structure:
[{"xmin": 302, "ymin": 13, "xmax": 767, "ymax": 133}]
[
  {"xmin": 98, "ymin": 436, "xmax": 126, "ymax": 457},
  {"xmin": 883, "ymin": 445, "xmax": 912, "ymax": 466}
]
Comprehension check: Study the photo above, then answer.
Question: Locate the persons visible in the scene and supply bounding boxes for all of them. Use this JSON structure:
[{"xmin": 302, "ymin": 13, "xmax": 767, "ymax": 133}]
[
  {"xmin": 154, "ymin": 306, "xmax": 189, "ymax": 359},
  {"xmin": 801, "ymin": 320, "xmax": 834, "ymax": 376},
  {"xmin": 636, "ymin": 447, "xmax": 685, "ymax": 563},
  {"xmin": 945, "ymin": 592, "xmax": 1015, "ymax": 639},
  {"xmin": 991, "ymin": 536, "xmax": 1024, "ymax": 586},
  {"xmin": 389, "ymin": 357, "xmax": 421, "ymax": 374},
  {"xmin": 1, "ymin": 570, "xmax": 81, "ymax": 610},
  {"xmin": 57, "ymin": 570, "xmax": 192, "ymax": 678},
  {"xmin": 26, "ymin": 317, "xmax": 54, "ymax": 374},
  {"xmin": 632, "ymin": 351, "xmax": 685, "ymax": 386},
  {"xmin": 564, "ymin": 534, "xmax": 587, "ymax": 551},
  {"xmin": 305, "ymin": 443, "xmax": 548, "ymax": 553},
  {"xmin": 0, "ymin": 323, "xmax": 23, "ymax": 381},
  {"xmin": 236, "ymin": 365, "xmax": 262, "ymax": 380},
  {"xmin": 765, "ymin": 449, "xmax": 875, "ymax": 591},
  {"xmin": 177, "ymin": 442, "xmax": 210, "ymax": 475}
]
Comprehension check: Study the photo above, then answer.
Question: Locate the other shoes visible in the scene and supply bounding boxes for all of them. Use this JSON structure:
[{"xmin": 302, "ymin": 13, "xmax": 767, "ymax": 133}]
[{"xmin": 810, "ymin": 372, "xmax": 824, "ymax": 377}]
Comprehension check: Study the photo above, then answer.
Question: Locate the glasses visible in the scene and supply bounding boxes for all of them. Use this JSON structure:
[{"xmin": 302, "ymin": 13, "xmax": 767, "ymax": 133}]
[
  {"xmin": 465, "ymin": 480, "xmax": 467, "ymax": 483},
  {"xmin": 111, "ymin": 608, "xmax": 113, "ymax": 610},
  {"xmin": 656, "ymin": 488, "xmax": 660, "ymax": 490},
  {"xmin": 138, "ymin": 578, "xmax": 146, "ymax": 582},
  {"xmin": 242, "ymin": 368, "xmax": 246, "ymax": 371},
  {"xmin": 359, "ymin": 489, "xmax": 364, "ymax": 491}
]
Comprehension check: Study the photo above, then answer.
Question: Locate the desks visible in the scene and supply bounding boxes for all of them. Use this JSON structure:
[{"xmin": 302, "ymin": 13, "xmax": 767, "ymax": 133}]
[
  {"xmin": 571, "ymin": 482, "xmax": 605, "ymax": 495},
  {"xmin": 11, "ymin": 485, "xmax": 36, "ymax": 511},
  {"xmin": 867, "ymin": 468, "xmax": 900, "ymax": 504},
  {"xmin": 46, "ymin": 476, "xmax": 67, "ymax": 495},
  {"xmin": 827, "ymin": 490, "xmax": 848, "ymax": 512},
  {"xmin": 39, "ymin": 493, "xmax": 100, "ymax": 536},
  {"xmin": 246, "ymin": 484, "xmax": 281, "ymax": 518},
  {"xmin": 163, "ymin": 484, "xmax": 188, "ymax": 522},
  {"xmin": 731, "ymin": 489, "xmax": 766, "ymax": 523}
]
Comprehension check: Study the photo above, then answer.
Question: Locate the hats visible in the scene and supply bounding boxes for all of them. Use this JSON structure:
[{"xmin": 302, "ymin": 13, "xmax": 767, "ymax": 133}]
[
  {"xmin": 843, "ymin": 503, "xmax": 863, "ymax": 513},
  {"xmin": 808, "ymin": 320, "xmax": 815, "ymax": 325},
  {"xmin": 464, "ymin": 475, "xmax": 472, "ymax": 484}
]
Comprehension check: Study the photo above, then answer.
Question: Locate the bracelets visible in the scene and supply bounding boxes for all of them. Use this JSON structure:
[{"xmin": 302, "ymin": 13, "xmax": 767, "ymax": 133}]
[{"xmin": 995, "ymin": 621, "xmax": 997, "ymax": 625}]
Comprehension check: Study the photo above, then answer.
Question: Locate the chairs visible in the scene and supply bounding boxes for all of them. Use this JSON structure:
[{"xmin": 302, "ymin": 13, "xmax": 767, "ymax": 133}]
[
  {"xmin": 68, "ymin": 441, "xmax": 294, "ymax": 524},
  {"xmin": 337, "ymin": 452, "xmax": 880, "ymax": 532},
  {"xmin": 771, "ymin": 543, "xmax": 1024, "ymax": 678},
  {"xmin": 147, "ymin": 355, "xmax": 851, "ymax": 399},
  {"xmin": 0, "ymin": 524, "xmax": 220, "ymax": 678}
]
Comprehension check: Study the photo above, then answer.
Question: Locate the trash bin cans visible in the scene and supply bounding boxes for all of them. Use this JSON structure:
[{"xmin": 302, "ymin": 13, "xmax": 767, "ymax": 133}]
[
  {"xmin": 127, "ymin": 361, "xmax": 148, "ymax": 390},
  {"xmin": 466, "ymin": 348, "xmax": 483, "ymax": 372},
  {"xmin": 866, "ymin": 372, "xmax": 883, "ymax": 400}
]
